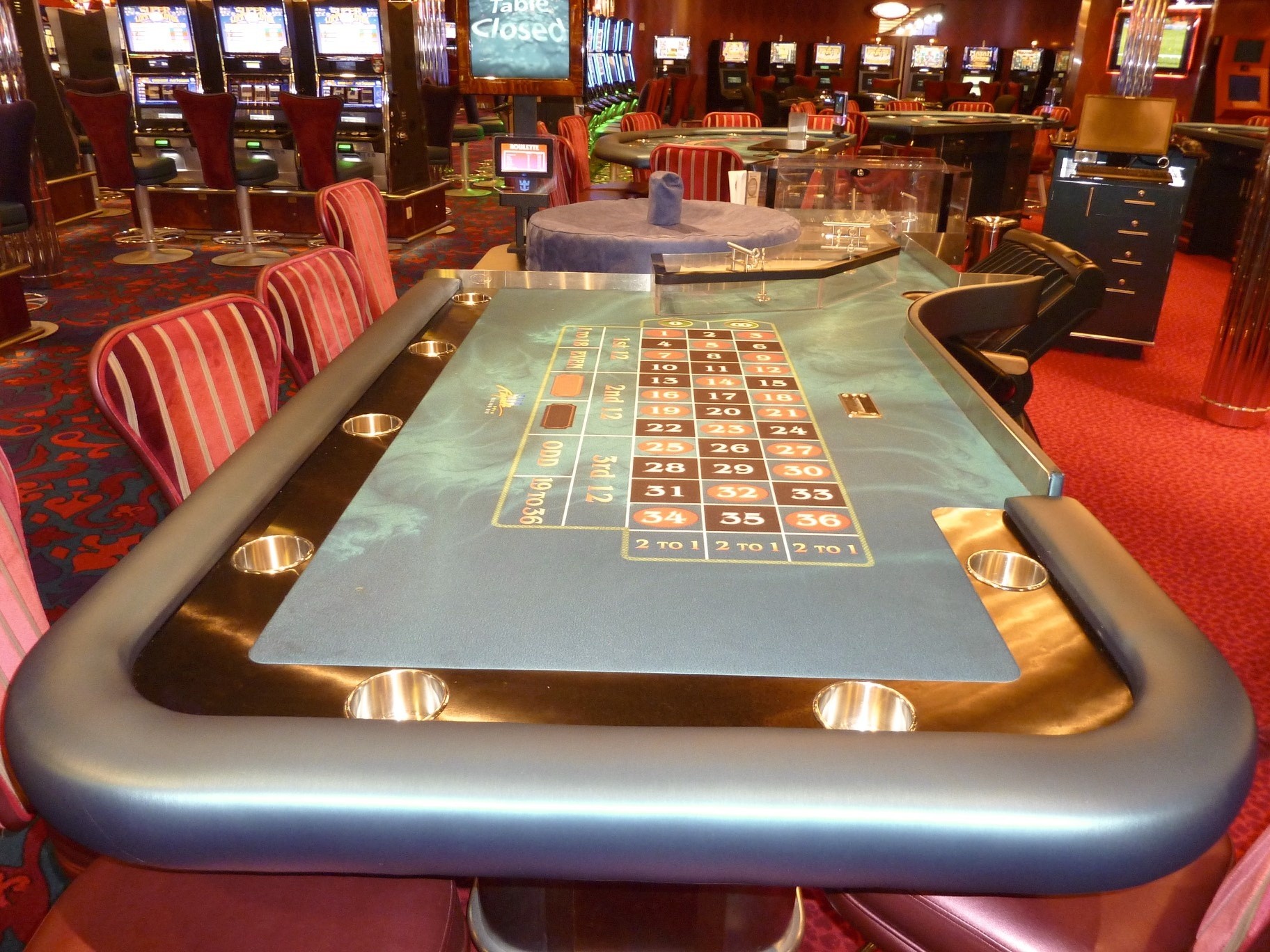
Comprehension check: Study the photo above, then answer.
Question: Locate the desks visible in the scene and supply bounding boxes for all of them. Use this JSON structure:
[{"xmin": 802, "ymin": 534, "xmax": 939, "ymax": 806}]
[
  {"xmin": 525, "ymin": 200, "xmax": 800, "ymax": 274},
  {"xmin": 863, "ymin": 111, "xmax": 1065, "ymax": 227},
  {"xmin": 595, "ymin": 127, "xmax": 858, "ymax": 188},
  {"xmin": 3, "ymin": 232, "xmax": 1258, "ymax": 897}
]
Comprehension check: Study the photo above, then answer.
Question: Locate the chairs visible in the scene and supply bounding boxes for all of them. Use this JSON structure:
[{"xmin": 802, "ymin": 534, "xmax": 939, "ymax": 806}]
[
  {"xmin": 0, "ymin": 421, "xmax": 1270, "ymax": 952},
  {"xmin": 174, "ymin": 88, "xmax": 291, "ymax": 267},
  {"xmin": 943, "ymin": 228, "xmax": 1106, "ymax": 448},
  {"xmin": 422, "ymin": 78, "xmax": 453, "ymax": 178},
  {"xmin": 65, "ymin": 90, "xmax": 193, "ymax": 264},
  {"xmin": 255, "ymin": 257, "xmax": 374, "ymax": 394},
  {"xmin": 537, "ymin": 76, "xmax": 1069, "ymax": 211},
  {"xmin": 281, "ymin": 93, "xmax": 374, "ymax": 248},
  {"xmin": 91, "ymin": 293, "xmax": 284, "ymax": 517},
  {"xmin": 315, "ymin": 178, "xmax": 398, "ymax": 322}
]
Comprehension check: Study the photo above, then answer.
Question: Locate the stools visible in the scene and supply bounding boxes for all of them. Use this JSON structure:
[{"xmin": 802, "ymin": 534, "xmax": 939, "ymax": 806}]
[{"xmin": 445, "ymin": 116, "xmax": 506, "ymax": 197}]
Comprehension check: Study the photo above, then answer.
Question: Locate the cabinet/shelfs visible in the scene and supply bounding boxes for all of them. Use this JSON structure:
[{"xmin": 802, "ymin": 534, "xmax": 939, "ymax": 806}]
[{"xmin": 1041, "ymin": 145, "xmax": 1198, "ymax": 359}]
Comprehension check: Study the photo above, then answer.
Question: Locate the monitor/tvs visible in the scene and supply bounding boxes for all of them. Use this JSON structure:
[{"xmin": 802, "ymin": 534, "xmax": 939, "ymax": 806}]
[
  {"xmin": 132, "ymin": 73, "xmax": 198, "ymax": 107},
  {"xmin": 1073, "ymin": 93, "xmax": 1178, "ymax": 168},
  {"xmin": 212, "ymin": 0, "xmax": 290, "ymax": 58},
  {"xmin": 813, "ymin": 43, "xmax": 844, "ymax": 64},
  {"xmin": 316, "ymin": 74, "xmax": 385, "ymax": 110},
  {"xmin": 307, "ymin": 0, "xmax": 384, "ymax": 57},
  {"xmin": 911, "ymin": 45, "xmax": 949, "ymax": 68},
  {"xmin": 861, "ymin": 44, "xmax": 896, "ymax": 66},
  {"xmin": 963, "ymin": 47, "xmax": 999, "ymax": 73},
  {"xmin": 653, "ymin": 36, "xmax": 691, "ymax": 59},
  {"xmin": 225, "ymin": 73, "xmax": 291, "ymax": 110},
  {"xmin": 117, "ymin": 0, "xmax": 196, "ymax": 56},
  {"xmin": 1111, "ymin": 13, "xmax": 1191, "ymax": 71},
  {"xmin": 494, "ymin": 137, "xmax": 553, "ymax": 177},
  {"xmin": 769, "ymin": 42, "xmax": 797, "ymax": 63},
  {"xmin": 1011, "ymin": 48, "xmax": 1044, "ymax": 73},
  {"xmin": 720, "ymin": 40, "xmax": 750, "ymax": 63}
]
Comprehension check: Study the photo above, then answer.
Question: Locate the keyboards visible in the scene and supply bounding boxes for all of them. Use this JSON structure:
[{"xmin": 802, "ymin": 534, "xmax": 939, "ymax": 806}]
[{"xmin": 1076, "ymin": 164, "xmax": 1174, "ymax": 183}]
